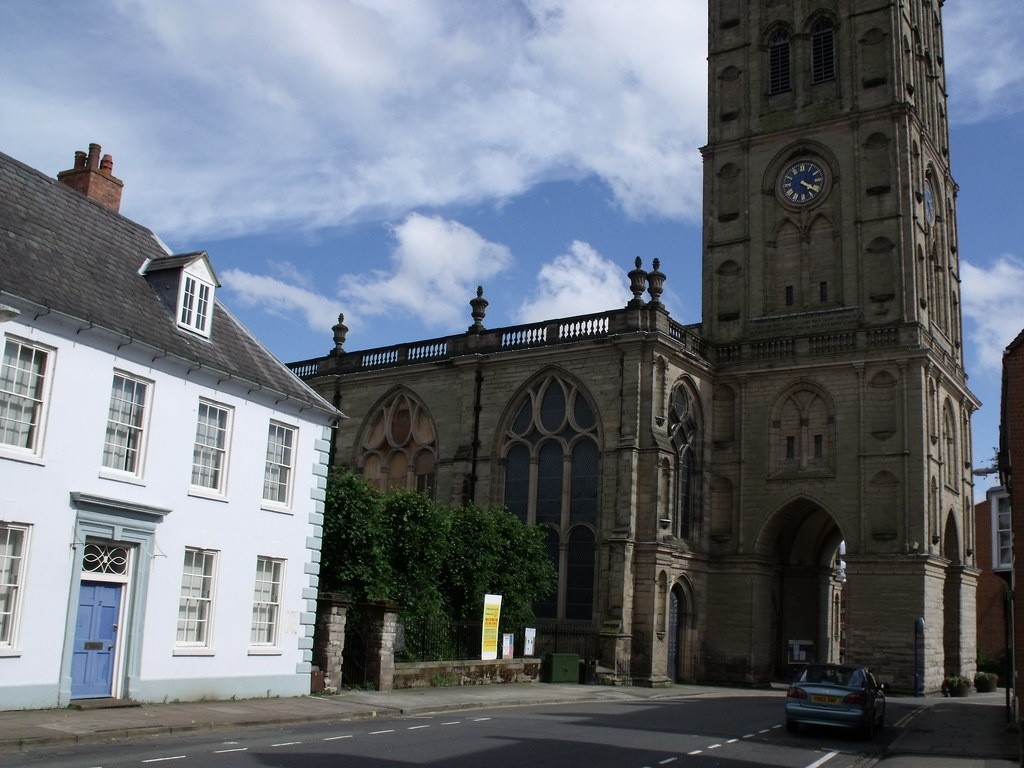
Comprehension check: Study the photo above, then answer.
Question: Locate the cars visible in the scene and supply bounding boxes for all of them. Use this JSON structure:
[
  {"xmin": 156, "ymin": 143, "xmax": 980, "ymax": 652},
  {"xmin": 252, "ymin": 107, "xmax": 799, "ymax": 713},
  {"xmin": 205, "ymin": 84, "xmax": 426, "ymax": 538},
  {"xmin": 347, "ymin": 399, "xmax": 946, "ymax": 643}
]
[{"xmin": 785, "ymin": 663, "xmax": 886, "ymax": 742}]
